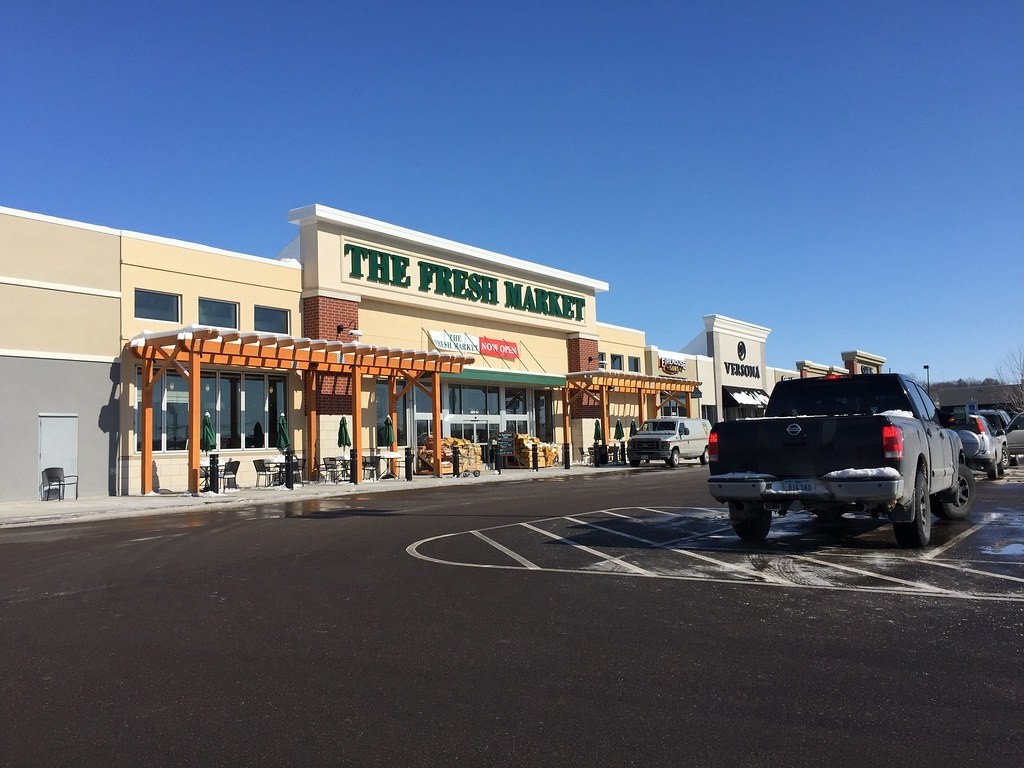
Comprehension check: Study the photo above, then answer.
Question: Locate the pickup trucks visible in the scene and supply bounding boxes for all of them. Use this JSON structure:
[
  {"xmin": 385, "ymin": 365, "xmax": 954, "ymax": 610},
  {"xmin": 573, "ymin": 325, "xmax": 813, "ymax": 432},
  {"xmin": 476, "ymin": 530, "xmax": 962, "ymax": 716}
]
[{"xmin": 707, "ymin": 373, "xmax": 978, "ymax": 548}]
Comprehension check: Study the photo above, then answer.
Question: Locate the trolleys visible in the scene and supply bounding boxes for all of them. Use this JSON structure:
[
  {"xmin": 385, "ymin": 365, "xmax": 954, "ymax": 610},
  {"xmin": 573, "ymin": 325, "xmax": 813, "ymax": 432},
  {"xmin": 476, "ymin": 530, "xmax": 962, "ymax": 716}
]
[{"xmin": 459, "ymin": 447, "xmax": 481, "ymax": 477}]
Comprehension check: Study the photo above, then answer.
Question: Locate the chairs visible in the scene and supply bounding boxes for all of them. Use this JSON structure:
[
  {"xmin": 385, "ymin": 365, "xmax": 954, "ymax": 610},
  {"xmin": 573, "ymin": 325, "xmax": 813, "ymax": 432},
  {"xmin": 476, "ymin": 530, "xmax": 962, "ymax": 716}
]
[
  {"xmin": 394, "ymin": 461, "xmax": 405, "ymax": 480},
  {"xmin": 188, "ymin": 463, "xmax": 210, "ymax": 488},
  {"xmin": 44, "ymin": 467, "xmax": 79, "ymax": 502},
  {"xmin": 219, "ymin": 461, "xmax": 240, "ymax": 493},
  {"xmin": 578, "ymin": 446, "xmax": 627, "ymax": 466},
  {"xmin": 253, "ymin": 456, "xmax": 381, "ymax": 487}
]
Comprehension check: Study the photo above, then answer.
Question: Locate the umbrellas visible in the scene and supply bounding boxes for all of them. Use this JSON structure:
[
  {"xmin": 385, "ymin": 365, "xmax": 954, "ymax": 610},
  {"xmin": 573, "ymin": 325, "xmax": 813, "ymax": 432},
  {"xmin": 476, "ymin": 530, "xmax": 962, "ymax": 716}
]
[
  {"xmin": 383, "ymin": 415, "xmax": 395, "ymax": 477},
  {"xmin": 201, "ymin": 410, "xmax": 217, "ymax": 488},
  {"xmin": 339, "ymin": 414, "xmax": 351, "ymax": 479},
  {"xmin": 630, "ymin": 418, "xmax": 638, "ymax": 437},
  {"xmin": 614, "ymin": 418, "xmax": 624, "ymax": 443},
  {"xmin": 277, "ymin": 413, "xmax": 291, "ymax": 458},
  {"xmin": 594, "ymin": 418, "xmax": 603, "ymax": 444}
]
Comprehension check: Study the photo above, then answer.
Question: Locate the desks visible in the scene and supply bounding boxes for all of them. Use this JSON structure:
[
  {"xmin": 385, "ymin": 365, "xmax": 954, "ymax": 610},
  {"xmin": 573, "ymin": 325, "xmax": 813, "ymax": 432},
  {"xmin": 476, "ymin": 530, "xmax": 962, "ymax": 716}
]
[
  {"xmin": 200, "ymin": 464, "xmax": 226, "ymax": 487},
  {"xmin": 265, "ymin": 461, "xmax": 298, "ymax": 487},
  {"xmin": 329, "ymin": 458, "xmax": 350, "ymax": 480},
  {"xmin": 380, "ymin": 456, "xmax": 402, "ymax": 478}
]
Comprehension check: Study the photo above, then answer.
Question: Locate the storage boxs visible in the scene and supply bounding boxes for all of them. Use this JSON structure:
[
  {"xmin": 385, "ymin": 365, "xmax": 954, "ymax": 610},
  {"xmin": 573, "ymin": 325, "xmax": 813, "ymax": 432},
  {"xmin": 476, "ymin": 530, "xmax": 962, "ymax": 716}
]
[{"xmin": 515, "ymin": 433, "xmax": 546, "ymax": 468}]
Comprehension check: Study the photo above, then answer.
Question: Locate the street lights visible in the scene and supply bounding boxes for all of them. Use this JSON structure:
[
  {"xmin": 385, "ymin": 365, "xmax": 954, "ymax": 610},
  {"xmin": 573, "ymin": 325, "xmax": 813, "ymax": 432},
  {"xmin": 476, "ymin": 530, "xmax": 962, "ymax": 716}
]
[{"xmin": 923, "ymin": 364, "xmax": 930, "ymax": 395}]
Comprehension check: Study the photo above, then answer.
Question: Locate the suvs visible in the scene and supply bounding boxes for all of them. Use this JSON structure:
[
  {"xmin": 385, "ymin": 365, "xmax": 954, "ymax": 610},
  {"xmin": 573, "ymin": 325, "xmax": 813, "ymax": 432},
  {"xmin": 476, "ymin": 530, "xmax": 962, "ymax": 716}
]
[
  {"xmin": 624, "ymin": 416, "xmax": 712, "ymax": 468},
  {"xmin": 1004, "ymin": 410, "xmax": 1024, "ymax": 455},
  {"xmin": 977, "ymin": 408, "xmax": 1011, "ymax": 429},
  {"xmin": 946, "ymin": 412, "xmax": 1007, "ymax": 480}
]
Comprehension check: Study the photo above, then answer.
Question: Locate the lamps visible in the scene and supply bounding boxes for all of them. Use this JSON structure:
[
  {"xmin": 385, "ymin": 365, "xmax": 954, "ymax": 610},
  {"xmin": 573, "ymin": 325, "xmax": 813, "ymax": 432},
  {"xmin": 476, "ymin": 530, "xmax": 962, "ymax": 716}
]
[
  {"xmin": 588, "ymin": 353, "xmax": 609, "ymax": 365},
  {"xmin": 337, "ymin": 319, "xmax": 363, "ymax": 337}
]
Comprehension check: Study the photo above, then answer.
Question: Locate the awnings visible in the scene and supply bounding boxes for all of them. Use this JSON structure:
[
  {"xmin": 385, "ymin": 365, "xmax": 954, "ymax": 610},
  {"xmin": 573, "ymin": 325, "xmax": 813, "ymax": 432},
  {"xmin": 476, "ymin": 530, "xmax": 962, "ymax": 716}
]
[{"xmin": 721, "ymin": 386, "xmax": 771, "ymax": 409}]
